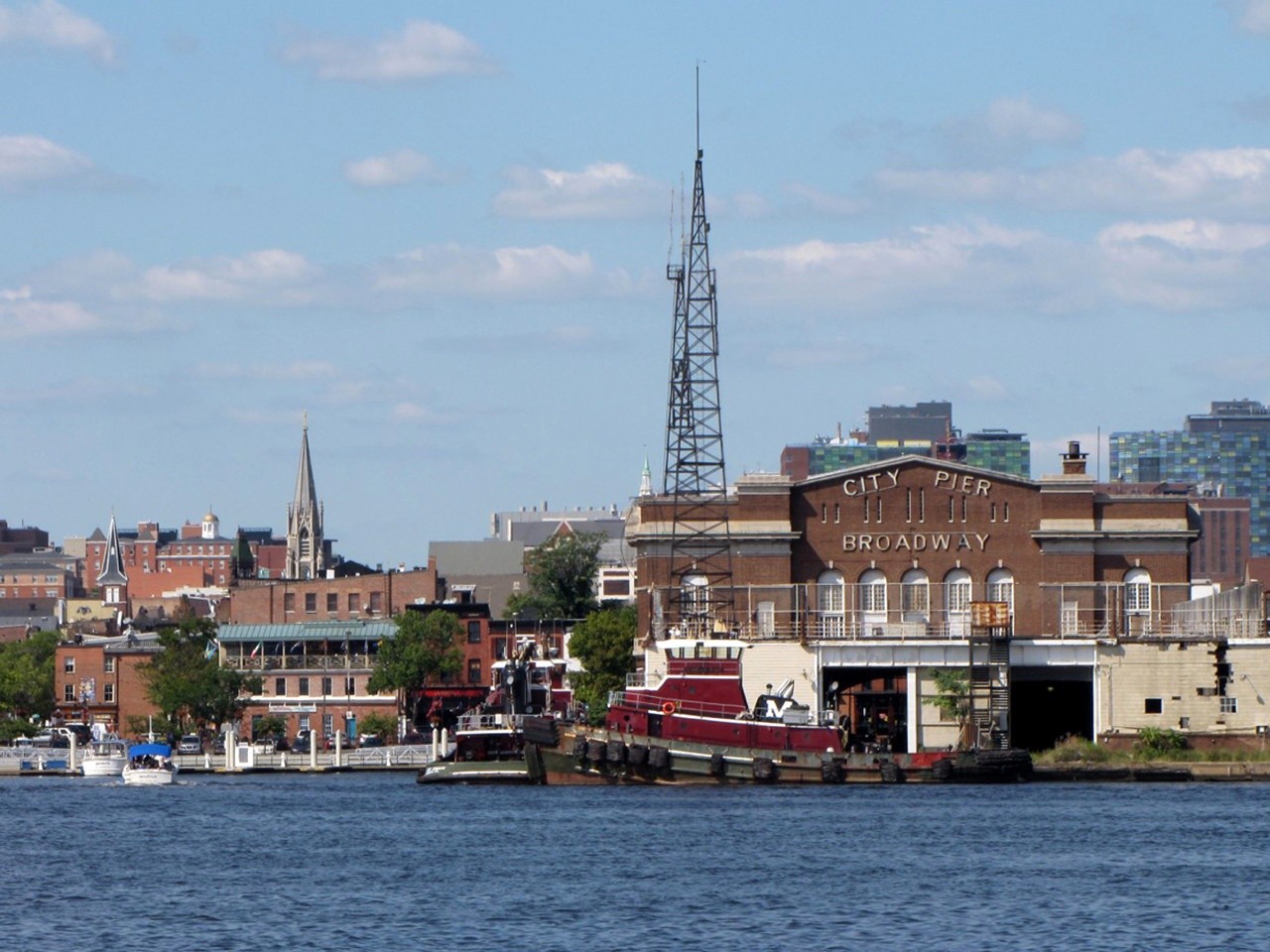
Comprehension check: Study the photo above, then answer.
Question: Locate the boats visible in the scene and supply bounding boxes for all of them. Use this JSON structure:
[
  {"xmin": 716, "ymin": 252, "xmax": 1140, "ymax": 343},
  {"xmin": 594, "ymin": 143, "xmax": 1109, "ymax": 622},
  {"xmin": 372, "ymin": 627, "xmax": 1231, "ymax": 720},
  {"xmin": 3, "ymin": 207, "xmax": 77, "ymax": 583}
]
[
  {"xmin": 78, "ymin": 738, "xmax": 135, "ymax": 778},
  {"xmin": 513, "ymin": 631, "xmax": 1035, "ymax": 789},
  {"xmin": 417, "ymin": 628, "xmax": 585, "ymax": 787},
  {"xmin": 120, "ymin": 742, "xmax": 180, "ymax": 785}
]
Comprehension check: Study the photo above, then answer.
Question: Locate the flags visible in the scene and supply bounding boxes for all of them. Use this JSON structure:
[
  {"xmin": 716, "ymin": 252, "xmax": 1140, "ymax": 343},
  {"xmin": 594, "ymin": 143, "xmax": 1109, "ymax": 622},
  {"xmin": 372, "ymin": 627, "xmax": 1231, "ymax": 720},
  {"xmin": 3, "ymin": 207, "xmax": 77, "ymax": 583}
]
[
  {"xmin": 203, "ymin": 639, "xmax": 219, "ymax": 660},
  {"xmin": 249, "ymin": 631, "xmax": 386, "ymax": 660}
]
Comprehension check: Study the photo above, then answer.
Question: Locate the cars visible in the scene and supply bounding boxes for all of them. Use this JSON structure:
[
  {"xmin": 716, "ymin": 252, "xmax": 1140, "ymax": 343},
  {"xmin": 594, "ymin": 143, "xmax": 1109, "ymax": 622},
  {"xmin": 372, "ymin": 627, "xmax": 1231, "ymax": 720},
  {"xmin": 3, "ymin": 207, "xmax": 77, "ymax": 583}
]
[
  {"xmin": 252, "ymin": 738, "xmax": 276, "ymax": 755},
  {"xmin": 324, "ymin": 732, "xmax": 349, "ymax": 749},
  {"xmin": 358, "ymin": 729, "xmax": 387, "ymax": 748},
  {"xmin": 289, "ymin": 734, "xmax": 310, "ymax": 754},
  {"xmin": 401, "ymin": 726, "xmax": 433, "ymax": 744},
  {"xmin": 49, "ymin": 724, "xmax": 98, "ymax": 748},
  {"xmin": 214, "ymin": 743, "xmax": 225, "ymax": 755},
  {"xmin": 178, "ymin": 734, "xmax": 205, "ymax": 755}
]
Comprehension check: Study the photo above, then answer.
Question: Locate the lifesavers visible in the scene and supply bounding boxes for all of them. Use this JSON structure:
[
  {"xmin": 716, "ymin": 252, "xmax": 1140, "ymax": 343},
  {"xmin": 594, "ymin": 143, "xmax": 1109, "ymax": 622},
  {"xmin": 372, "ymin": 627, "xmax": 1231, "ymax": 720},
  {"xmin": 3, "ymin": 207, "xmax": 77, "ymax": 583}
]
[{"xmin": 662, "ymin": 701, "xmax": 675, "ymax": 715}]
